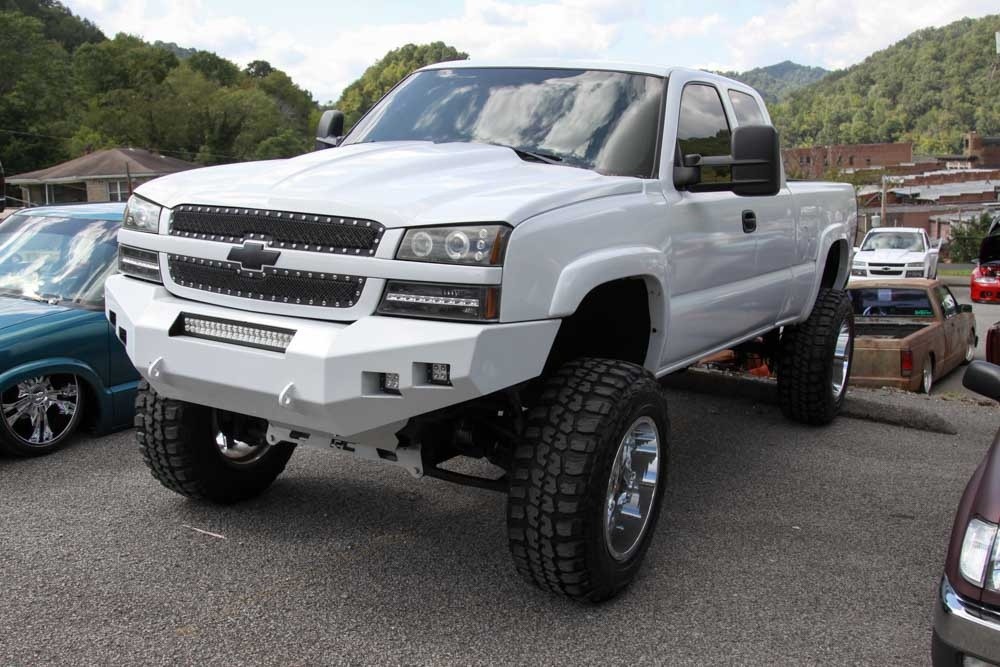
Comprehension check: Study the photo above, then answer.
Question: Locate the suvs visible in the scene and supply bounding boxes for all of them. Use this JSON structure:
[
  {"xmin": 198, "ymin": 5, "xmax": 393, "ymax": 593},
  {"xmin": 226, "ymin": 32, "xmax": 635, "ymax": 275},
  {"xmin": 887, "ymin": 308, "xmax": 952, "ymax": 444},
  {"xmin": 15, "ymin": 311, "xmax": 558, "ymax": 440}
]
[{"xmin": 98, "ymin": 59, "xmax": 869, "ymax": 604}]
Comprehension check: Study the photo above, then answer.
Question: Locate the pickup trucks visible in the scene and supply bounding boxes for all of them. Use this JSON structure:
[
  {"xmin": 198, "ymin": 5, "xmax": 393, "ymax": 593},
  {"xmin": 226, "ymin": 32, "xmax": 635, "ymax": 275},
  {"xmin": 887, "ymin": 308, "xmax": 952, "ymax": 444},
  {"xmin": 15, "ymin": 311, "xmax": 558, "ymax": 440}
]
[{"xmin": 841, "ymin": 279, "xmax": 980, "ymax": 393}]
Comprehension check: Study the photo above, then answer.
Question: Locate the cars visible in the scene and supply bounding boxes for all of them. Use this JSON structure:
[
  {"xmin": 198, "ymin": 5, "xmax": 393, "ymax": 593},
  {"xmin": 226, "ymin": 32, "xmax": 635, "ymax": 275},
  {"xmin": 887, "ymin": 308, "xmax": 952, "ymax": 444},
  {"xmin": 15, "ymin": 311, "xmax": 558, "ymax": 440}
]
[
  {"xmin": 970, "ymin": 233, "xmax": 1000, "ymax": 304},
  {"xmin": 930, "ymin": 359, "xmax": 999, "ymax": 667},
  {"xmin": 0, "ymin": 199, "xmax": 145, "ymax": 457},
  {"xmin": 848, "ymin": 225, "xmax": 940, "ymax": 283}
]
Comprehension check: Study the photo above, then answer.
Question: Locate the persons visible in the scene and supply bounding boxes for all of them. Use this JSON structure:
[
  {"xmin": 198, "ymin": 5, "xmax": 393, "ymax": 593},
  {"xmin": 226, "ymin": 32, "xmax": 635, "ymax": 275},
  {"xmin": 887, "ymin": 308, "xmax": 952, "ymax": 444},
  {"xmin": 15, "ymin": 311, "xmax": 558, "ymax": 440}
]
[{"xmin": 695, "ymin": 329, "xmax": 781, "ymax": 370}]
